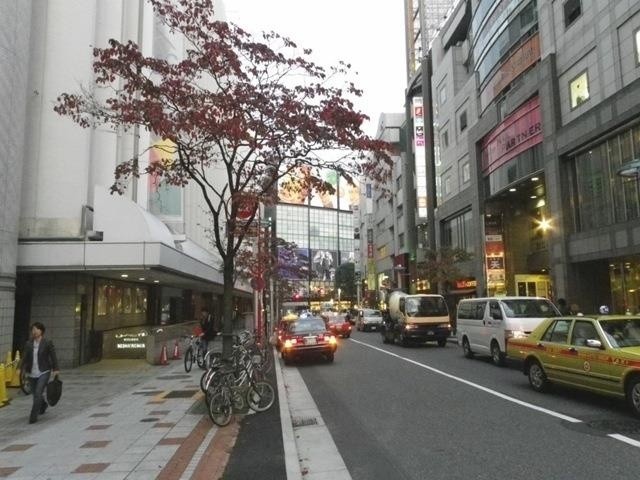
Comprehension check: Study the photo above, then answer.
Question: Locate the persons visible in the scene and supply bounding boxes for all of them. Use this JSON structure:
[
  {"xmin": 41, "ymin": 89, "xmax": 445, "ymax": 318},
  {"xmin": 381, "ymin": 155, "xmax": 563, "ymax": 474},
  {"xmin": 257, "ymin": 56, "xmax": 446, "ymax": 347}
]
[
  {"xmin": 525, "ymin": 302, "xmax": 538, "ymax": 317},
  {"xmin": 553, "ymin": 298, "xmax": 569, "ymax": 317},
  {"xmin": 199, "ymin": 307, "xmax": 217, "ymax": 354},
  {"xmin": 15, "ymin": 323, "xmax": 60, "ymax": 424}
]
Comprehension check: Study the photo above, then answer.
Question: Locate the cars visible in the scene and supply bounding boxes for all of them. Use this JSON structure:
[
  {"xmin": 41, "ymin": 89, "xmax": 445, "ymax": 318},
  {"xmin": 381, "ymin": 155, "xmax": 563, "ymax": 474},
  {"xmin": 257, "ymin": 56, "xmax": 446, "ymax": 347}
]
[
  {"xmin": 280, "ymin": 316, "xmax": 338, "ymax": 366},
  {"xmin": 273, "ymin": 307, "xmax": 388, "ymax": 349},
  {"xmin": 503, "ymin": 310, "xmax": 640, "ymax": 416}
]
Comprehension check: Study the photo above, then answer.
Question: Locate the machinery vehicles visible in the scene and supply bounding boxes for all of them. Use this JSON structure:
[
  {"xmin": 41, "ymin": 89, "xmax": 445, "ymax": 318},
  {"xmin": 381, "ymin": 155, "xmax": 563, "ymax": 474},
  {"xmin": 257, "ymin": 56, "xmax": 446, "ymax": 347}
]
[{"xmin": 374, "ymin": 283, "xmax": 454, "ymax": 350}]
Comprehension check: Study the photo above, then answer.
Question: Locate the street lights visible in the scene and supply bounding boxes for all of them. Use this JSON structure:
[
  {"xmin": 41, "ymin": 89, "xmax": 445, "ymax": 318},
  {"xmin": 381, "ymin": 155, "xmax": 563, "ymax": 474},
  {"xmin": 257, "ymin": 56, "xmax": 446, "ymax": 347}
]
[
  {"xmin": 616, "ymin": 158, "xmax": 640, "ymax": 192},
  {"xmin": 355, "ymin": 281, "xmax": 363, "ymax": 308},
  {"xmin": 393, "ymin": 263, "xmax": 407, "ymax": 290}
]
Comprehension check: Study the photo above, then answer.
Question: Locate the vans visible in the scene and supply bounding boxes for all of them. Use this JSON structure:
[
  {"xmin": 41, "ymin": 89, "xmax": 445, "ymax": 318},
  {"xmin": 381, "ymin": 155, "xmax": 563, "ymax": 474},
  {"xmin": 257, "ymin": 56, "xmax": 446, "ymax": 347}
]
[{"xmin": 454, "ymin": 295, "xmax": 565, "ymax": 365}]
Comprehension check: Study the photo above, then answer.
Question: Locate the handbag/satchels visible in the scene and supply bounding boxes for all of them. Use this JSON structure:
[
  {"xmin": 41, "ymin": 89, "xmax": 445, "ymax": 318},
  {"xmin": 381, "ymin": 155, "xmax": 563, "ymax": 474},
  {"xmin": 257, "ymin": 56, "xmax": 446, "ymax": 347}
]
[{"xmin": 47, "ymin": 375, "xmax": 61, "ymax": 406}]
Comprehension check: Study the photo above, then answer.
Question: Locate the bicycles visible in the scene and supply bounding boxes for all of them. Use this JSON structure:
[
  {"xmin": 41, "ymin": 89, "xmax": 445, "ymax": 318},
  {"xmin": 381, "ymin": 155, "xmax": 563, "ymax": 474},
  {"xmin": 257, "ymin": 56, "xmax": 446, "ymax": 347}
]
[
  {"xmin": 181, "ymin": 332, "xmax": 206, "ymax": 374},
  {"xmin": 199, "ymin": 329, "xmax": 277, "ymax": 427},
  {"xmin": 20, "ymin": 366, "xmax": 32, "ymax": 395}
]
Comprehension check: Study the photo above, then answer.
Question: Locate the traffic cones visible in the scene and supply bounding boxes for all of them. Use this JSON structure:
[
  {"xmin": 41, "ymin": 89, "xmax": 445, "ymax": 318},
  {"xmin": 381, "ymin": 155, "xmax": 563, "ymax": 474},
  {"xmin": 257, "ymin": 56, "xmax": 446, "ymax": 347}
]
[
  {"xmin": 172, "ymin": 340, "xmax": 181, "ymax": 360},
  {"xmin": 0, "ymin": 349, "xmax": 21, "ymax": 407},
  {"xmin": 157, "ymin": 341, "xmax": 171, "ymax": 366}
]
